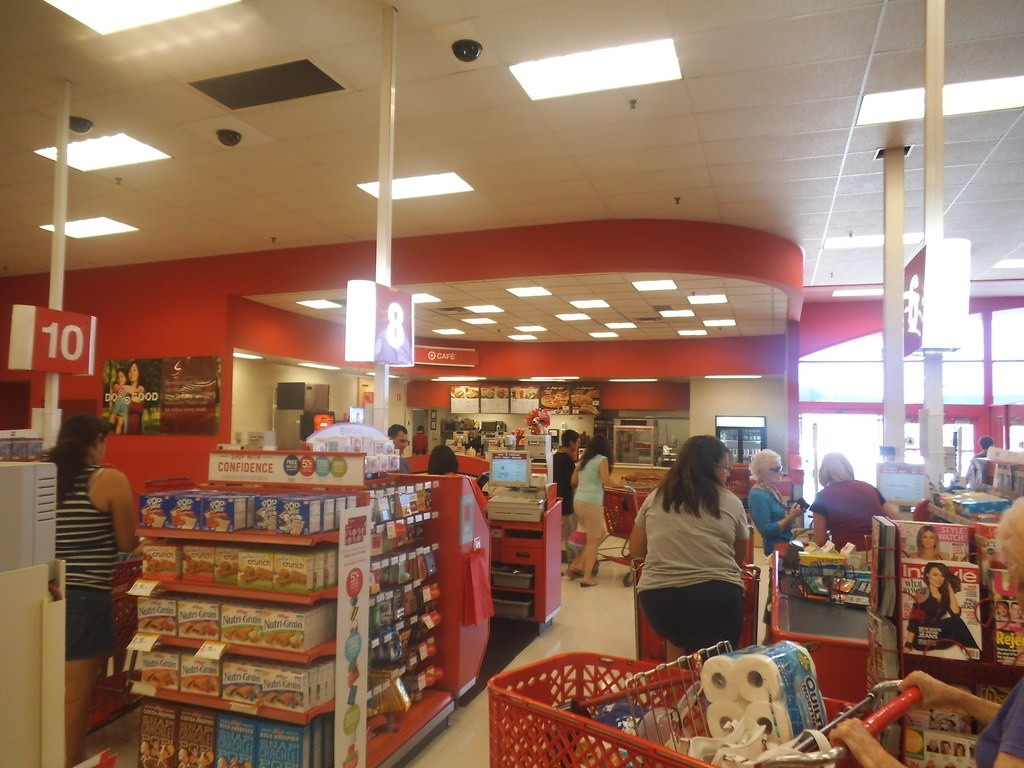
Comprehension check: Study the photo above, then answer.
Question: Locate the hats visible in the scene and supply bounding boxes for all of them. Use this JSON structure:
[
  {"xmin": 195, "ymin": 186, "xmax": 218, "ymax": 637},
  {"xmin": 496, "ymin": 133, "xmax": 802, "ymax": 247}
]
[{"xmin": 417, "ymin": 425, "xmax": 425, "ymax": 434}]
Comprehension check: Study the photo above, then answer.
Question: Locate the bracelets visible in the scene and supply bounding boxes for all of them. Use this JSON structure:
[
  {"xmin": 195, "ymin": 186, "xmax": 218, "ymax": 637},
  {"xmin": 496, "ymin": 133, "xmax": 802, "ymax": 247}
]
[{"xmin": 904, "ymin": 642, "xmax": 912, "ymax": 648}]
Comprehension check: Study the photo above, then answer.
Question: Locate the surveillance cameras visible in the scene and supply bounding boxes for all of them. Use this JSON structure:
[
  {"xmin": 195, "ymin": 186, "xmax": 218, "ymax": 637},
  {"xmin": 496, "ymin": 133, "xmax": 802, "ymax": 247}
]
[
  {"xmin": 218, "ymin": 132, "xmax": 241, "ymax": 146},
  {"xmin": 69, "ymin": 118, "xmax": 92, "ymax": 134},
  {"xmin": 451, "ymin": 39, "xmax": 482, "ymax": 63}
]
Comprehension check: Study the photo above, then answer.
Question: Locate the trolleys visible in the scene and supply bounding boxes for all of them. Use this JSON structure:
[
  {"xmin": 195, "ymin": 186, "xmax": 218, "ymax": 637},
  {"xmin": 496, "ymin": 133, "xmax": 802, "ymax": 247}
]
[
  {"xmin": 593, "ymin": 476, "xmax": 666, "ymax": 590},
  {"xmin": 486, "ymin": 635, "xmax": 922, "ymax": 768},
  {"xmin": 629, "ymin": 558, "xmax": 762, "ymax": 670}
]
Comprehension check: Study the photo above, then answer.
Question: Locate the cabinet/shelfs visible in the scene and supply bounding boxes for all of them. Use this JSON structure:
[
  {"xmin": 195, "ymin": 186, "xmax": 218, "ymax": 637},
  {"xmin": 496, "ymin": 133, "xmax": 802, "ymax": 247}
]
[{"xmin": 125, "ymin": 479, "xmax": 373, "ymax": 768}]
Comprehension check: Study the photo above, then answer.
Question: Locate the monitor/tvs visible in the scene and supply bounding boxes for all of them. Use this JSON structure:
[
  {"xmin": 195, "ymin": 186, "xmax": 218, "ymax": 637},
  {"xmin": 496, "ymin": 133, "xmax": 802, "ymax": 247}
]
[
  {"xmin": 876, "ymin": 463, "xmax": 929, "ymax": 506},
  {"xmin": 489, "ymin": 450, "xmax": 531, "ymax": 488}
]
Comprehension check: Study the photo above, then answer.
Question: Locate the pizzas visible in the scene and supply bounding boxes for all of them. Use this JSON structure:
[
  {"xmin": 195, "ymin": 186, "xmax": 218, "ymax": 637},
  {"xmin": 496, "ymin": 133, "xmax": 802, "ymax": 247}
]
[
  {"xmin": 541, "ymin": 394, "xmax": 568, "ymax": 408},
  {"xmin": 571, "ymin": 394, "xmax": 593, "ymax": 405}
]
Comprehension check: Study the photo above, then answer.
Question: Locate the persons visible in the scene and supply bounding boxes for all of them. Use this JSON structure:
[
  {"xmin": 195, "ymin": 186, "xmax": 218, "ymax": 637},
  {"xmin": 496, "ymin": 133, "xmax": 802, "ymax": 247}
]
[
  {"xmin": 110, "ymin": 361, "xmax": 145, "ymax": 434},
  {"xmin": 413, "ymin": 425, "xmax": 429, "ymax": 457},
  {"xmin": 1011, "ymin": 602, "xmax": 1024, "ymax": 622},
  {"xmin": 748, "ymin": 449, "xmax": 803, "ymax": 646},
  {"xmin": 996, "ymin": 600, "xmax": 1009, "ymax": 620},
  {"xmin": 572, "ymin": 434, "xmax": 628, "ymax": 588},
  {"xmin": 925, "ymin": 739, "xmax": 965, "ymax": 757},
  {"xmin": 630, "ymin": 435, "xmax": 750, "ymax": 667},
  {"xmin": 428, "ymin": 445, "xmax": 488, "ymax": 512},
  {"xmin": 909, "ymin": 526, "xmax": 950, "ymax": 560},
  {"xmin": 809, "ymin": 453, "xmax": 900, "ymax": 554},
  {"xmin": 553, "ymin": 430, "xmax": 581, "ymax": 564},
  {"xmin": 829, "ymin": 493, "xmax": 1024, "ymax": 768},
  {"xmin": 965, "ymin": 436, "xmax": 994, "ymax": 485},
  {"xmin": 387, "ymin": 424, "xmax": 409, "ymax": 474},
  {"xmin": 903, "ymin": 563, "xmax": 981, "ymax": 654},
  {"xmin": 43, "ymin": 413, "xmax": 140, "ymax": 768}
]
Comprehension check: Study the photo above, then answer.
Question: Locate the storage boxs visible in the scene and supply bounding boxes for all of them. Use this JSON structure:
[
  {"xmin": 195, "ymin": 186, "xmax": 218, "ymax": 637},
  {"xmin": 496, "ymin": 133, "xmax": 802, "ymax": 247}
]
[
  {"xmin": 320, "ymin": 496, "xmax": 335, "ymax": 533},
  {"xmin": 202, "ymin": 496, "xmax": 247, "ymax": 531},
  {"xmin": 492, "ymin": 570, "xmax": 533, "ymax": 590},
  {"xmin": 252, "ymin": 495, "xmax": 277, "ymax": 531},
  {"xmin": 275, "ymin": 497, "xmax": 322, "ymax": 535},
  {"xmin": 491, "ymin": 597, "xmax": 534, "ymax": 617},
  {"xmin": 132, "ymin": 541, "xmax": 346, "ymax": 768},
  {"xmin": 797, "ymin": 551, "xmax": 848, "ymax": 567},
  {"xmin": 136, "ymin": 494, "xmax": 170, "ymax": 528},
  {"xmin": 334, "ymin": 496, "xmax": 347, "ymax": 531},
  {"xmin": 169, "ymin": 494, "xmax": 201, "ymax": 529},
  {"xmin": 799, "ymin": 565, "xmax": 846, "ymax": 577},
  {"xmin": 246, "ymin": 495, "xmax": 256, "ymax": 529},
  {"xmin": 347, "ymin": 495, "xmax": 358, "ymax": 508}
]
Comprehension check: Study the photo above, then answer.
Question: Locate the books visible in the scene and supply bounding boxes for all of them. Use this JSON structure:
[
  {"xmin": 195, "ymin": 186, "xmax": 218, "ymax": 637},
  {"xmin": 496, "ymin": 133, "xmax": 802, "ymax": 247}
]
[{"xmin": 868, "ymin": 515, "xmax": 1024, "ymax": 679}]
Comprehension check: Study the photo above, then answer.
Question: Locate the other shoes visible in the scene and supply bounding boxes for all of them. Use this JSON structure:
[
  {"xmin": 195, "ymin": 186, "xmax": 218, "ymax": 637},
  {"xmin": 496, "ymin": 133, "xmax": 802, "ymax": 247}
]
[
  {"xmin": 580, "ymin": 579, "xmax": 598, "ymax": 587},
  {"xmin": 569, "ymin": 565, "xmax": 583, "ymax": 575}
]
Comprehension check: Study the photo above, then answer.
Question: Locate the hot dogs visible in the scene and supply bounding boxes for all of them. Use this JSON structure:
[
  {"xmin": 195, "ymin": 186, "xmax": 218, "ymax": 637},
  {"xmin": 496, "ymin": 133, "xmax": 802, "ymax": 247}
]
[
  {"xmin": 455, "ymin": 390, "xmax": 464, "ymax": 398},
  {"xmin": 580, "ymin": 404, "xmax": 597, "ymax": 413}
]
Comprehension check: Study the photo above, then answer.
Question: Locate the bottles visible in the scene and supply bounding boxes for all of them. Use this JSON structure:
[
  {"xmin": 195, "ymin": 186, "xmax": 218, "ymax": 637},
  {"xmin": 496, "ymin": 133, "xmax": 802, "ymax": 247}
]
[{"xmin": 721, "ymin": 429, "xmax": 761, "ymax": 464}]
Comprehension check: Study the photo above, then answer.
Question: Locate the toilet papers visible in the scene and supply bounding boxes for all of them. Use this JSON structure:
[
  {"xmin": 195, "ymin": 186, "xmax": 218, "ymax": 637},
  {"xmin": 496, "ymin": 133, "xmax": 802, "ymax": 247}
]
[{"xmin": 700, "ymin": 640, "xmax": 829, "ymax": 749}]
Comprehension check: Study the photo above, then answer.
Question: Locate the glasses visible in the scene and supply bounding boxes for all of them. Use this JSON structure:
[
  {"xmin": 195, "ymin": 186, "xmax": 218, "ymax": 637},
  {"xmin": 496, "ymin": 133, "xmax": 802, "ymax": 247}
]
[
  {"xmin": 397, "ymin": 439, "xmax": 411, "ymax": 447},
  {"xmin": 767, "ymin": 465, "xmax": 783, "ymax": 472},
  {"xmin": 711, "ymin": 461, "xmax": 732, "ymax": 473}
]
